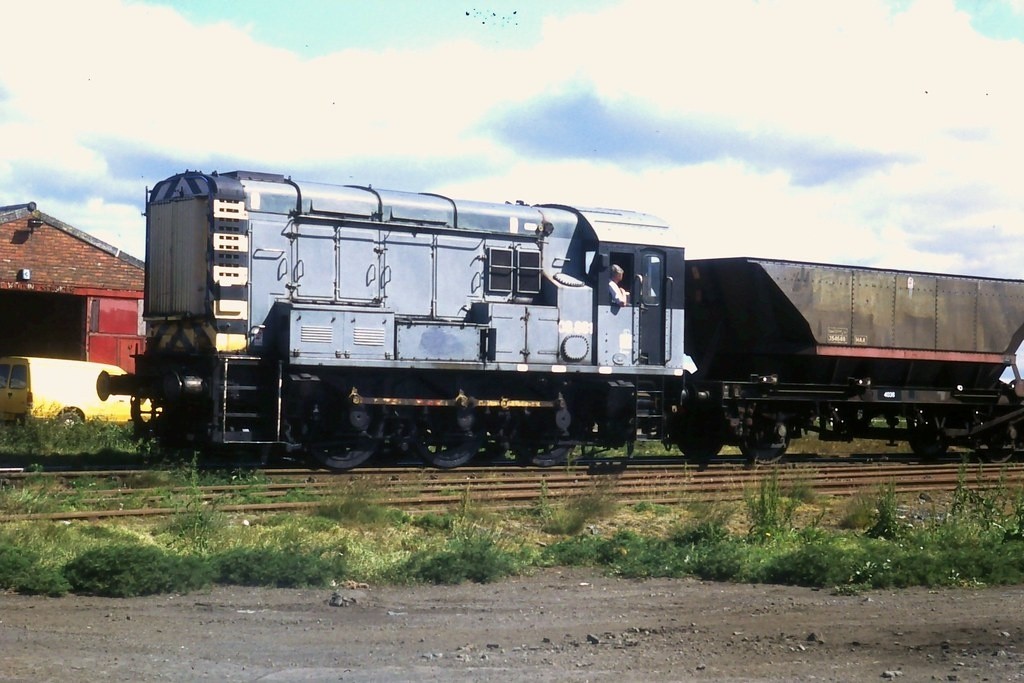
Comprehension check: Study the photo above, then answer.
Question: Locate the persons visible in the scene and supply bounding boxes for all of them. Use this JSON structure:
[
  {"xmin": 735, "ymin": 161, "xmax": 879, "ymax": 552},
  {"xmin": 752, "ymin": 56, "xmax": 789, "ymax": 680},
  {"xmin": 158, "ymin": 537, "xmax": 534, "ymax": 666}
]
[{"xmin": 609, "ymin": 263, "xmax": 626, "ymax": 307}]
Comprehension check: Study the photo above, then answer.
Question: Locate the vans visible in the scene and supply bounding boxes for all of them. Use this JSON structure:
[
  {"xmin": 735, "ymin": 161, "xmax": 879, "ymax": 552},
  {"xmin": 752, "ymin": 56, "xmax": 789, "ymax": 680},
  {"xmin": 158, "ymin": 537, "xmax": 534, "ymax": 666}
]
[{"xmin": 0, "ymin": 355, "xmax": 160, "ymax": 427}]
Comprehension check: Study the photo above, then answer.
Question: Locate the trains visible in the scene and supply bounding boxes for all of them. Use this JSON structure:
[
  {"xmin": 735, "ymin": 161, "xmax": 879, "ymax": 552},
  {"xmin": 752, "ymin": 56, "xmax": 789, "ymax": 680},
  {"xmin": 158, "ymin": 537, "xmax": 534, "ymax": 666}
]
[{"xmin": 96, "ymin": 167, "xmax": 1024, "ymax": 474}]
